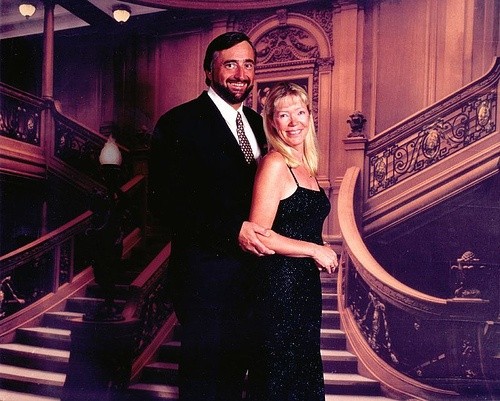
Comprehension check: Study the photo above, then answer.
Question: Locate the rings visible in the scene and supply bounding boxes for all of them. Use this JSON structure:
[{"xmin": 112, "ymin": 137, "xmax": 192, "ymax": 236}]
[{"xmin": 331, "ymin": 266, "xmax": 335, "ymax": 269}]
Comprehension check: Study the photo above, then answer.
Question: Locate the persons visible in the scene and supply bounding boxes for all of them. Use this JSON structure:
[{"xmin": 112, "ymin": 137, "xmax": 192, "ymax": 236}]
[
  {"xmin": 240, "ymin": 80, "xmax": 340, "ymax": 400},
  {"xmin": 141, "ymin": 30, "xmax": 278, "ymax": 401}
]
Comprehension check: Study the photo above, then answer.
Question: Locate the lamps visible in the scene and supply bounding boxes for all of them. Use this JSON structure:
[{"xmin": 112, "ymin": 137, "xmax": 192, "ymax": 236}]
[
  {"xmin": 97, "ymin": 134, "xmax": 125, "ymax": 195},
  {"xmin": 17, "ymin": 0, "xmax": 37, "ymax": 20},
  {"xmin": 111, "ymin": 3, "xmax": 133, "ymax": 25}
]
